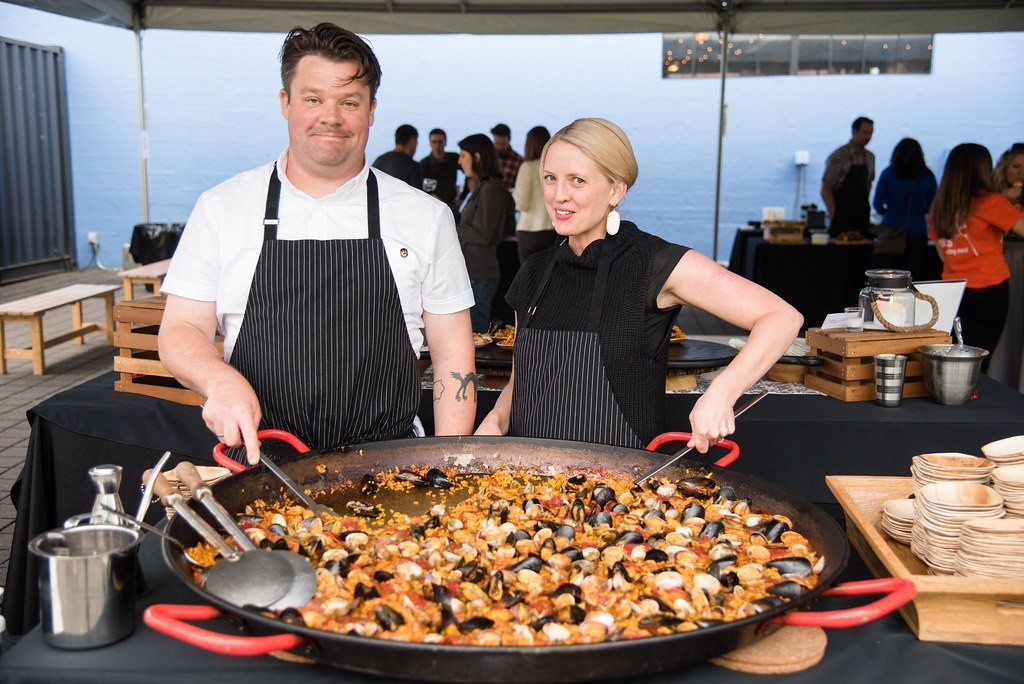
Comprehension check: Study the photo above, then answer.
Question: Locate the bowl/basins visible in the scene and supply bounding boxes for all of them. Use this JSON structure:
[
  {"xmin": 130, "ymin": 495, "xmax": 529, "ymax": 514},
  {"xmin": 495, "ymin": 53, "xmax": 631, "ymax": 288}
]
[{"xmin": 916, "ymin": 343, "xmax": 990, "ymax": 407}]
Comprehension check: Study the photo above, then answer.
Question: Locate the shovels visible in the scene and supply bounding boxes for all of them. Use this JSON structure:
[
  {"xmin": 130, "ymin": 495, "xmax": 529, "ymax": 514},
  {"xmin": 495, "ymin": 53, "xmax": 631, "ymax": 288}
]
[
  {"xmin": 633, "ymin": 388, "xmax": 769, "ymax": 485},
  {"xmin": 199, "ymin": 401, "xmax": 337, "ymax": 519}
]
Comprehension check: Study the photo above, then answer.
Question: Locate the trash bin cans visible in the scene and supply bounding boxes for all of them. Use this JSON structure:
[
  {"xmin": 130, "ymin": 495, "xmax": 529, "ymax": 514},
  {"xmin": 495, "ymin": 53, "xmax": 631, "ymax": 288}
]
[{"xmin": 134, "ymin": 223, "xmax": 185, "ymax": 291}]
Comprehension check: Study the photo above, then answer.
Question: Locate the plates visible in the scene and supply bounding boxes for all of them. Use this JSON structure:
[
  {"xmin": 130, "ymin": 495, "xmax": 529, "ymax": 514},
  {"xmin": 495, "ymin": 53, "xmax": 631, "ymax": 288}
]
[
  {"xmin": 475, "ymin": 336, "xmax": 514, "ymax": 351},
  {"xmin": 670, "ymin": 338, "xmax": 688, "ymax": 344},
  {"xmin": 881, "ymin": 434, "xmax": 1024, "ymax": 579}
]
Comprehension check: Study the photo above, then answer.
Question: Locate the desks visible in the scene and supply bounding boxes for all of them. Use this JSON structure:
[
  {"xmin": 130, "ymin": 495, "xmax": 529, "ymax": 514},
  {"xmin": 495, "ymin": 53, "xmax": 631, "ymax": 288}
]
[
  {"xmin": 726, "ymin": 228, "xmax": 938, "ymax": 333},
  {"xmin": 117, "ymin": 258, "xmax": 170, "ymax": 325}
]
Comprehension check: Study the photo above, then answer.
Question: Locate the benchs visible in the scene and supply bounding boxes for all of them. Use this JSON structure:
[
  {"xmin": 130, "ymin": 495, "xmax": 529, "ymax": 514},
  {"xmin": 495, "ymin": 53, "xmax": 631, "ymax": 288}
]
[{"xmin": 0, "ymin": 284, "xmax": 122, "ymax": 375}]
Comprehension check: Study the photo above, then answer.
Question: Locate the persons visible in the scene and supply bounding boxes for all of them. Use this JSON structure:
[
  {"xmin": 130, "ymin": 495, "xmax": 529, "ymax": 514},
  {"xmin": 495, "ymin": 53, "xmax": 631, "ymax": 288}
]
[
  {"xmin": 987, "ymin": 143, "xmax": 1024, "ymax": 397},
  {"xmin": 455, "ymin": 134, "xmax": 506, "ymax": 336},
  {"xmin": 820, "ymin": 117, "xmax": 875, "ymax": 238},
  {"xmin": 872, "ymin": 138, "xmax": 937, "ymax": 281},
  {"xmin": 473, "ymin": 118, "xmax": 805, "ymax": 454},
  {"xmin": 372, "ymin": 125, "xmax": 421, "ymax": 189},
  {"xmin": 420, "ymin": 129, "xmax": 470, "ymax": 218},
  {"xmin": 927, "ymin": 143, "xmax": 1024, "ymax": 375},
  {"xmin": 511, "ymin": 126, "xmax": 557, "ymax": 265},
  {"xmin": 490, "ymin": 124, "xmax": 524, "ymax": 237},
  {"xmin": 158, "ymin": 21, "xmax": 477, "ymax": 472}
]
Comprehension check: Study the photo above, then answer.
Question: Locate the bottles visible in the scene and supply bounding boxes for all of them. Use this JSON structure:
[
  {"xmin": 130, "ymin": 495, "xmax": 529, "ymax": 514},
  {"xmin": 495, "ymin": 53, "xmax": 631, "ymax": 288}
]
[
  {"xmin": 88, "ymin": 464, "xmax": 124, "ymax": 527},
  {"xmin": 858, "ymin": 270, "xmax": 940, "ymax": 334}
]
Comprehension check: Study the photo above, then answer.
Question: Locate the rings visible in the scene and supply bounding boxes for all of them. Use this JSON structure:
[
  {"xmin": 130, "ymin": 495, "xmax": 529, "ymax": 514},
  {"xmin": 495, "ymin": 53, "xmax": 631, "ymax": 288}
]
[{"xmin": 716, "ymin": 438, "xmax": 724, "ymax": 443}]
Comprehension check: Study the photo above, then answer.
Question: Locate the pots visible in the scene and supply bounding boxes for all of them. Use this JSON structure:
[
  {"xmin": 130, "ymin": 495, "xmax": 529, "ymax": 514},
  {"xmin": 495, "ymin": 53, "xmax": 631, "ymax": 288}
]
[{"xmin": 143, "ymin": 431, "xmax": 919, "ymax": 684}]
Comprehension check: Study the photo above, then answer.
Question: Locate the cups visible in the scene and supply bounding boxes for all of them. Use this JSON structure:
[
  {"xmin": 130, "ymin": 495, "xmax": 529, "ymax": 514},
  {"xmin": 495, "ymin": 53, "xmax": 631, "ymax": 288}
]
[
  {"xmin": 844, "ymin": 307, "xmax": 865, "ymax": 333},
  {"xmin": 873, "ymin": 353, "xmax": 907, "ymax": 408},
  {"xmin": 422, "ymin": 177, "xmax": 438, "ymax": 194},
  {"xmin": 27, "ymin": 523, "xmax": 141, "ymax": 652}
]
